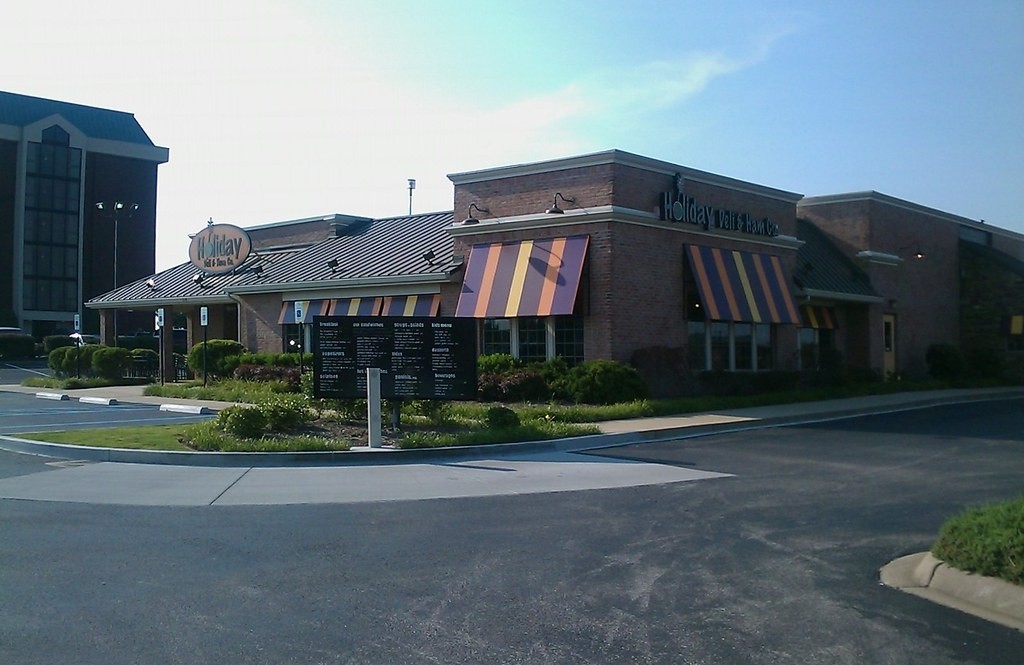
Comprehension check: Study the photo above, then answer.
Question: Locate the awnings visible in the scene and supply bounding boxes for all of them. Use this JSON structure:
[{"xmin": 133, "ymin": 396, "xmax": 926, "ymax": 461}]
[
  {"xmin": 278, "ymin": 300, "xmax": 329, "ymax": 323},
  {"xmin": 329, "ymin": 298, "xmax": 382, "ymax": 315},
  {"xmin": 381, "ymin": 296, "xmax": 441, "ymax": 317},
  {"xmin": 455, "ymin": 234, "xmax": 590, "ymax": 318},
  {"xmin": 684, "ymin": 243, "xmax": 804, "ymax": 324}
]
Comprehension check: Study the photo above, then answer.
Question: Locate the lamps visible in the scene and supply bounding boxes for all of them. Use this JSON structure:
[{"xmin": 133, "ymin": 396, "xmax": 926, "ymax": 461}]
[
  {"xmin": 146, "ymin": 279, "xmax": 156, "ymax": 292},
  {"xmin": 252, "ymin": 266, "xmax": 263, "ymax": 278},
  {"xmin": 423, "ymin": 251, "xmax": 436, "ymax": 265},
  {"xmin": 461, "ymin": 203, "xmax": 488, "ymax": 224},
  {"xmin": 193, "ymin": 275, "xmax": 205, "ymax": 288},
  {"xmin": 328, "ymin": 259, "xmax": 338, "ymax": 273},
  {"xmin": 545, "ymin": 192, "xmax": 575, "ymax": 215}
]
